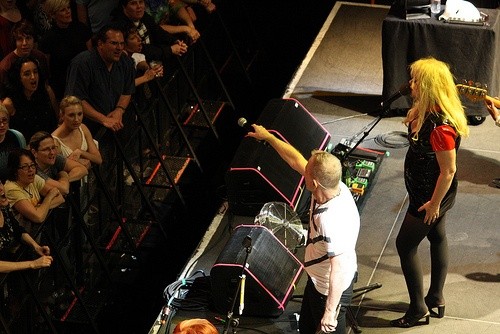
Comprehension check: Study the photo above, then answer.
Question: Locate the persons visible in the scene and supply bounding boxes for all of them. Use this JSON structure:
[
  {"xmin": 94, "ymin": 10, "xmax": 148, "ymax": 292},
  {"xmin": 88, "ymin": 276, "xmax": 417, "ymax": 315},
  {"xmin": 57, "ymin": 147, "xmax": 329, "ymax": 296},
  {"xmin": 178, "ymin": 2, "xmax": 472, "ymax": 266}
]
[
  {"xmin": 247, "ymin": 124, "xmax": 361, "ymax": 334},
  {"xmin": 390, "ymin": 57, "xmax": 469, "ymax": 328},
  {"xmin": 484, "ymin": 96, "xmax": 500, "ymax": 127},
  {"xmin": 0, "ymin": 0, "xmax": 225, "ymax": 334}
]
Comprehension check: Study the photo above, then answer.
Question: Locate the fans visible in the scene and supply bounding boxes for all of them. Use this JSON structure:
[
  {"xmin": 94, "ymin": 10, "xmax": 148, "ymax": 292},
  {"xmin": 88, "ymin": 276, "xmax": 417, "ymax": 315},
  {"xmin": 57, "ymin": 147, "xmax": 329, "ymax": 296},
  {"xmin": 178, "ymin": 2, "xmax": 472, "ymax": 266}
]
[{"xmin": 254, "ymin": 201, "xmax": 308, "ymax": 252}]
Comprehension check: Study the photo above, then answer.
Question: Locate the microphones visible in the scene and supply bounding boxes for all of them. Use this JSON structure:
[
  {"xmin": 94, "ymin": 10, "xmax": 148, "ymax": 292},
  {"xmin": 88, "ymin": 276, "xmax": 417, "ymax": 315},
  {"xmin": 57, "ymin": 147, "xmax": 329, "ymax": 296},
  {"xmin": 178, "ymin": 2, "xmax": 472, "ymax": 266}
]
[
  {"xmin": 237, "ymin": 117, "xmax": 255, "ymax": 133},
  {"xmin": 241, "ymin": 235, "xmax": 252, "ymax": 247}
]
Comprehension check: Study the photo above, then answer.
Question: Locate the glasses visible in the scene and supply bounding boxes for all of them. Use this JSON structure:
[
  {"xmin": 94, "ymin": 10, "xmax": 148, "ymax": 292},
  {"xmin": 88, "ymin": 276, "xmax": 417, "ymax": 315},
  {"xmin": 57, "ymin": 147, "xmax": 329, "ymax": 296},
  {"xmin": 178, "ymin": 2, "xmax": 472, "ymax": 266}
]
[
  {"xmin": 18, "ymin": 163, "xmax": 38, "ymax": 170},
  {"xmin": 39, "ymin": 146, "xmax": 59, "ymax": 152},
  {"xmin": 0, "ymin": 118, "xmax": 9, "ymax": 125}
]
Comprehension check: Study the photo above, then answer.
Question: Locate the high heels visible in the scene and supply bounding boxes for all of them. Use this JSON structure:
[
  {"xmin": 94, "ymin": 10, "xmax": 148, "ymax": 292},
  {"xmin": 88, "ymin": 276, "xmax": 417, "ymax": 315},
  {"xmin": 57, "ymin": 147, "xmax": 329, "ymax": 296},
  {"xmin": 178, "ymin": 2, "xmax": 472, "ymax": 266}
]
[
  {"xmin": 390, "ymin": 311, "xmax": 431, "ymax": 328},
  {"xmin": 424, "ymin": 294, "xmax": 446, "ymax": 318}
]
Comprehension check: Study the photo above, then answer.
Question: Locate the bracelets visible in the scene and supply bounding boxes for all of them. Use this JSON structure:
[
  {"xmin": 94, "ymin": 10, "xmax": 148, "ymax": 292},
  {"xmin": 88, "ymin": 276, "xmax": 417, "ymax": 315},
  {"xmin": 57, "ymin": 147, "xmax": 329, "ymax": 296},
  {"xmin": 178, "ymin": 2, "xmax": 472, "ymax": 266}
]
[
  {"xmin": 116, "ymin": 105, "xmax": 125, "ymax": 114},
  {"xmin": 185, "ymin": 5, "xmax": 191, "ymax": 9}
]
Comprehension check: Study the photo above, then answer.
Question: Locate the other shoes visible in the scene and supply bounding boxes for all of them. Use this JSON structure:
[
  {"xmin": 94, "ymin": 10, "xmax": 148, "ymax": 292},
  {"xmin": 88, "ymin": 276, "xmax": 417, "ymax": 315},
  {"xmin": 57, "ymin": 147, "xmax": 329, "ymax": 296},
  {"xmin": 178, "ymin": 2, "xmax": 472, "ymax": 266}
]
[
  {"xmin": 123, "ymin": 169, "xmax": 134, "ymax": 186},
  {"xmin": 492, "ymin": 179, "xmax": 500, "ymax": 187}
]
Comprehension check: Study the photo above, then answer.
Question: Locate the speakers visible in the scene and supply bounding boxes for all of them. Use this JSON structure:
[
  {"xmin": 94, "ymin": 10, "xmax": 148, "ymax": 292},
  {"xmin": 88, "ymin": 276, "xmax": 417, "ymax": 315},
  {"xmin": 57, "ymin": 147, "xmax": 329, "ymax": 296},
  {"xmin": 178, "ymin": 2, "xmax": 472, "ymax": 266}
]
[
  {"xmin": 211, "ymin": 225, "xmax": 306, "ymax": 318},
  {"xmin": 227, "ymin": 98, "xmax": 333, "ymax": 217}
]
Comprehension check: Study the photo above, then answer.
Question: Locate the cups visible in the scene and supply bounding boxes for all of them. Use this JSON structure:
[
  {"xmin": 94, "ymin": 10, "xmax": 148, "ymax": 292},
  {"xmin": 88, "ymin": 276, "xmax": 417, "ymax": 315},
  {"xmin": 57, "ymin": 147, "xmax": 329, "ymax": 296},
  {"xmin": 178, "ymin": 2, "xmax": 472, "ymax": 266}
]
[{"xmin": 430, "ymin": 0, "xmax": 441, "ymax": 14}]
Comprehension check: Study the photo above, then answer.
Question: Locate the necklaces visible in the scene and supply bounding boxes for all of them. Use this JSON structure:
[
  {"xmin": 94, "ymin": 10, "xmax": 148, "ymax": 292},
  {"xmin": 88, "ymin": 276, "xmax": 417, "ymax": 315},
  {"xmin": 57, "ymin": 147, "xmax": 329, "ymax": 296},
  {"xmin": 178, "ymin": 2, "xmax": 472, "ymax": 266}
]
[{"xmin": 23, "ymin": 188, "xmax": 33, "ymax": 198}]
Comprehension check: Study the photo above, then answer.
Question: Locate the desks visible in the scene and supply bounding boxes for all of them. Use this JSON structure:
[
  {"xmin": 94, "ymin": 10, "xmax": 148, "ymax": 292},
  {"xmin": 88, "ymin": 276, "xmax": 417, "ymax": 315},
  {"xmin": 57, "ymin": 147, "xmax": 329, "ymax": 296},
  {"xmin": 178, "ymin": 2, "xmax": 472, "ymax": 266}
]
[{"xmin": 381, "ymin": 4, "xmax": 500, "ymax": 119}]
